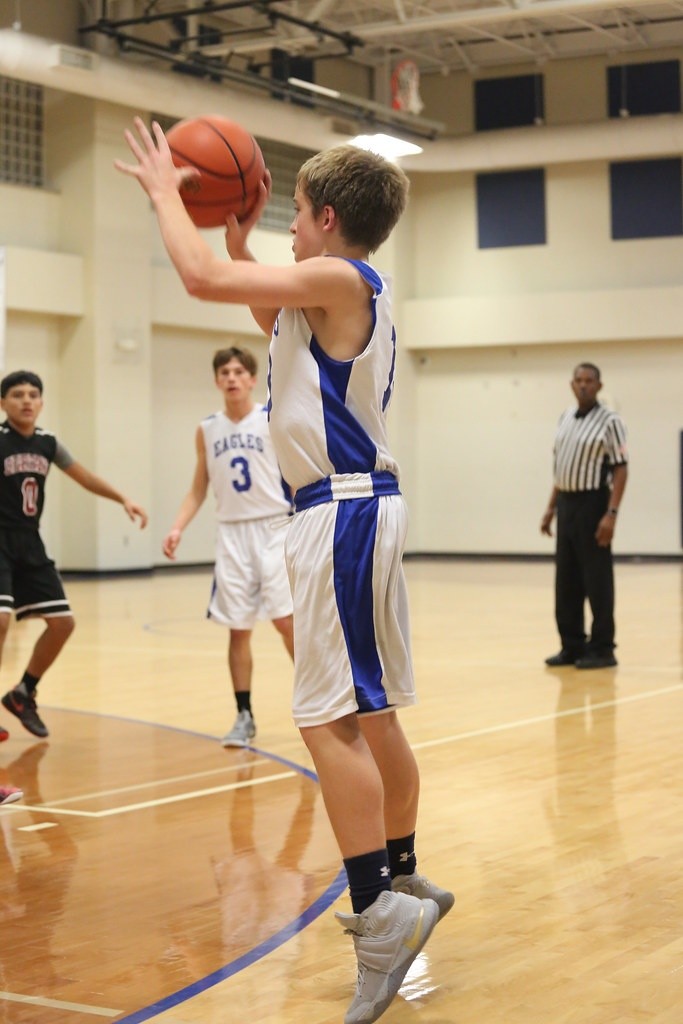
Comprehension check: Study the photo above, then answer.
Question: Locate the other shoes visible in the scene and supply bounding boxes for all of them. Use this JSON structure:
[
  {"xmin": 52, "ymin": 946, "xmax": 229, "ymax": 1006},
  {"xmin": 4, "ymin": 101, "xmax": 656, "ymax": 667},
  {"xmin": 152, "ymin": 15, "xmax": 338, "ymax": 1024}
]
[
  {"xmin": 545, "ymin": 646, "xmax": 581, "ymax": 664},
  {"xmin": 0, "ymin": 787, "xmax": 24, "ymax": 805},
  {"xmin": 575, "ymin": 650, "xmax": 617, "ymax": 668}
]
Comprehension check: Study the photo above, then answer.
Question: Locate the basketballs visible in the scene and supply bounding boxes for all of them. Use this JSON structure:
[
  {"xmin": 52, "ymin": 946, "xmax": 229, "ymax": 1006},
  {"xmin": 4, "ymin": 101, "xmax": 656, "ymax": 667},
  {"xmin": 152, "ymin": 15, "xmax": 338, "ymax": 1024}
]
[{"xmin": 160, "ymin": 112, "xmax": 267, "ymax": 229}]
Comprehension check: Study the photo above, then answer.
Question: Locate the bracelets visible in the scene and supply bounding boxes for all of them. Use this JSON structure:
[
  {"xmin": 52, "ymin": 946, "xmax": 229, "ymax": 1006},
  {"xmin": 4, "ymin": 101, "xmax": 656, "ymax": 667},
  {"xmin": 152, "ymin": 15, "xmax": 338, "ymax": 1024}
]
[{"xmin": 608, "ymin": 509, "xmax": 617, "ymax": 516}]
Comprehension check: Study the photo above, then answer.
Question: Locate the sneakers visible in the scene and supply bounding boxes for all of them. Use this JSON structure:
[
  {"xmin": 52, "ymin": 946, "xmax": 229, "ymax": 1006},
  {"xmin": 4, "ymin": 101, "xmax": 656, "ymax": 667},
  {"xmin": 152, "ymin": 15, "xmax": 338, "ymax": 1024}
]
[
  {"xmin": 388, "ymin": 868, "xmax": 454, "ymax": 921},
  {"xmin": 222, "ymin": 710, "xmax": 256, "ymax": 746},
  {"xmin": 335, "ymin": 887, "xmax": 440, "ymax": 1024},
  {"xmin": 1, "ymin": 689, "xmax": 48, "ymax": 737}
]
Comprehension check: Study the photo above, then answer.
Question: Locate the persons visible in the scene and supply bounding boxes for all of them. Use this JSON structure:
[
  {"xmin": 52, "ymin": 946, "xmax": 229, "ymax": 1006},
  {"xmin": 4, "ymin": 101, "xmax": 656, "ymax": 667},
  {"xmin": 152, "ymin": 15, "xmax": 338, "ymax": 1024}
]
[
  {"xmin": 113, "ymin": 117, "xmax": 456, "ymax": 1024},
  {"xmin": 170, "ymin": 746, "xmax": 317, "ymax": 1023},
  {"xmin": 0, "ymin": 371, "xmax": 149, "ymax": 743},
  {"xmin": 1, "ymin": 744, "xmax": 79, "ymax": 1024},
  {"xmin": 543, "ymin": 666, "xmax": 632, "ymax": 948},
  {"xmin": 541, "ymin": 364, "xmax": 628, "ymax": 668},
  {"xmin": 162, "ymin": 345, "xmax": 294, "ymax": 748}
]
